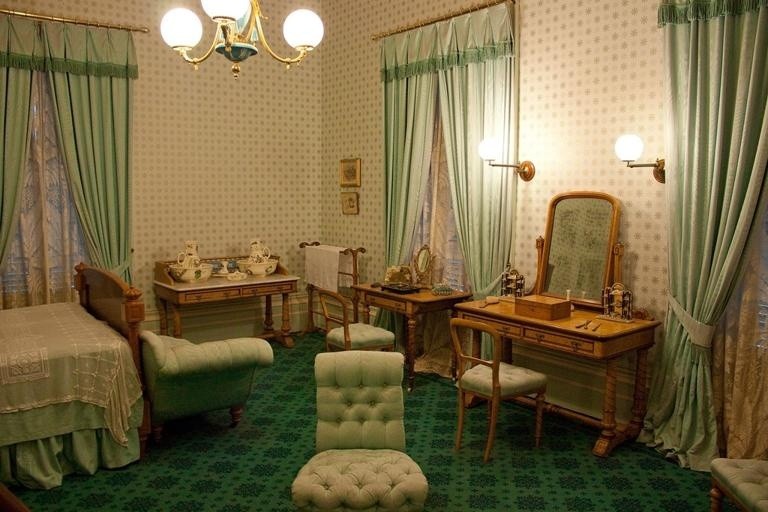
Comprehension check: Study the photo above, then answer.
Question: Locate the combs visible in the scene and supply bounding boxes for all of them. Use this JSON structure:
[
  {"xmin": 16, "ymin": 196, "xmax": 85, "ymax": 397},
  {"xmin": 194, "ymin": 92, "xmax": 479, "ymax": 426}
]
[{"xmin": 480, "ymin": 296, "xmax": 500, "ymax": 308}]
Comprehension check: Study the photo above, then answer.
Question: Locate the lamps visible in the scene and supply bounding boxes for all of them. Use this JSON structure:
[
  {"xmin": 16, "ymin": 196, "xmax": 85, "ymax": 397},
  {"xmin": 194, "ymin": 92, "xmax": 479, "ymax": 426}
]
[
  {"xmin": 613, "ymin": 134, "xmax": 664, "ymax": 185},
  {"xmin": 477, "ymin": 139, "xmax": 534, "ymax": 181},
  {"xmin": 160, "ymin": 0, "xmax": 324, "ymax": 81}
]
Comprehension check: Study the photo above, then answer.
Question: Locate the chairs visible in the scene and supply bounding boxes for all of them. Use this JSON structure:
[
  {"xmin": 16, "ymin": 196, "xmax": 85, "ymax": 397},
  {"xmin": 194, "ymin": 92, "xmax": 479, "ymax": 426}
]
[
  {"xmin": 138, "ymin": 328, "xmax": 273, "ymax": 445},
  {"xmin": 319, "ymin": 288, "xmax": 395, "ymax": 351},
  {"xmin": 450, "ymin": 318, "xmax": 548, "ymax": 462},
  {"xmin": 290, "ymin": 350, "xmax": 429, "ymax": 512}
]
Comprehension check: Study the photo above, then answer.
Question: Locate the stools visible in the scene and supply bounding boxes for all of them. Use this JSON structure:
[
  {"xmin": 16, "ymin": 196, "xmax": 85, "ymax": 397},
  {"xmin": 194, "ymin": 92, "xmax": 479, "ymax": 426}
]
[{"xmin": 709, "ymin": 458, "xmax": 768, "ymax": 512}]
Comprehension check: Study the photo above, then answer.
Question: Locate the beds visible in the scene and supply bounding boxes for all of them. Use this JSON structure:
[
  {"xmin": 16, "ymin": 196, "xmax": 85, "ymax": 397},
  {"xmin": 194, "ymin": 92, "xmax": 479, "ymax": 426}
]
[{"xmin": 0, "ymin": 263, "xmax": 152, "ymax": 491}]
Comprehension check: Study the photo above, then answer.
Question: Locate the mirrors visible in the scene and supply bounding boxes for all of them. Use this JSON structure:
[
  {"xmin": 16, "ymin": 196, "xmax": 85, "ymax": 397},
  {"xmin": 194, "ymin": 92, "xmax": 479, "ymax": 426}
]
[
  {"xmin": 525, "ymin": 191, "xmax": 624, "ymax": 310},
  {"xmin": 412, "ymin": 244, "xmax": 438, "ymax": 289}
]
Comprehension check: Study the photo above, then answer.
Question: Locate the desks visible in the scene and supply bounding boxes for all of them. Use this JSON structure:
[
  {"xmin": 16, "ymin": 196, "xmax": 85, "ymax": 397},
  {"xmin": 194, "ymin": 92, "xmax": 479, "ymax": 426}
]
[
  {"xmin": 350, "ymin": 284, "xmax": 472, "ymax": 394},
  {"xmin": 152, "ymin": 254, "xmax": 300, "ymax": 348},
  {"xmin": 454, "ymin": 294, "xmax": 663, "ymax": 457}
]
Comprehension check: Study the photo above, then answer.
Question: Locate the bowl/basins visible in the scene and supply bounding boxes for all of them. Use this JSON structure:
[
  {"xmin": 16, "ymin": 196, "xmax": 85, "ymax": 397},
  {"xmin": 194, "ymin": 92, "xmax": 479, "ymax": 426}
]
[
  {"xmin": 235, "ymin": 259, "xmax": 279, "ymax": 278},
  {"xmin": 164, "ymin": 262, "xmax": 213, "ymax": 283}
]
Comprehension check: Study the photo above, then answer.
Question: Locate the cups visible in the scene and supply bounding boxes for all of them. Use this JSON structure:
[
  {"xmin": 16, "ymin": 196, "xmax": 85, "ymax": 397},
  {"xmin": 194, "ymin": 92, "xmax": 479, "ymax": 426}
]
[{"xmin": 609, "ymin": 312, "xmax": 617, "ymax": 319}]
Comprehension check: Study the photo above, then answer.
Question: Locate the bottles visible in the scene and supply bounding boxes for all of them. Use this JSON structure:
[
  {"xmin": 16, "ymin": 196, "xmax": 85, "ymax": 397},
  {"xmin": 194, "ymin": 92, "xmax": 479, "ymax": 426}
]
[
  {"xmin": 219, "ymin": 259, "xmax": 230, "ymax": 274},
  {"xmin": 431, "ymin": 282, "xmax": 453, "ymax": 296}
]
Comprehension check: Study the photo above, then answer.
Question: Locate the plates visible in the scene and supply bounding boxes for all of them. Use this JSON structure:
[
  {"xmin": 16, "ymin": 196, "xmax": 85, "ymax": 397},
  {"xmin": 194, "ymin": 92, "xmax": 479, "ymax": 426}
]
[
  {"xmin": 212, "ymin": 271, "xmax": 237, "ymax": 278},
  {"xmin": 227, "ymin": 271, "xmax": 249, "ymax": 282}
]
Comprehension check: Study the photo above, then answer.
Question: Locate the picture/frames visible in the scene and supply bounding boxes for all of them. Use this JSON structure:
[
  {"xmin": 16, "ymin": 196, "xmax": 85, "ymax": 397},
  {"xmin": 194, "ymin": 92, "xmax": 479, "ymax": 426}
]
[
  {"xmin": 340, "ymin": 191, "xmax": 359, "ymax": 215},
  {"xmin": 339, "ymin": 158, "xmax": 361, "ymax": 188}
]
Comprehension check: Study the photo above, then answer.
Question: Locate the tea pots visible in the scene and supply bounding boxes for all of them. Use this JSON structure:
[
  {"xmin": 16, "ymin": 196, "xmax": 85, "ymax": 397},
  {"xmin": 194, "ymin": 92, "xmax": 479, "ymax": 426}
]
[
  {"xmin": 247, "ymin": 239, "xmax": 271, "ymax": 264},
  {"xmin": 177, "ymin": 239, "xmax": 202, "ymax": 269}
]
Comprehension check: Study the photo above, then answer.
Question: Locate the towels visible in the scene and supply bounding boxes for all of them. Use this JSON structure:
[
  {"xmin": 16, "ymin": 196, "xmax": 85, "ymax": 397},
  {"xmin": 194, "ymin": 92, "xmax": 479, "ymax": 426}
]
[{"xmin": 303, "ymin": 245, "xmax": 360, "ymax": 294}]
